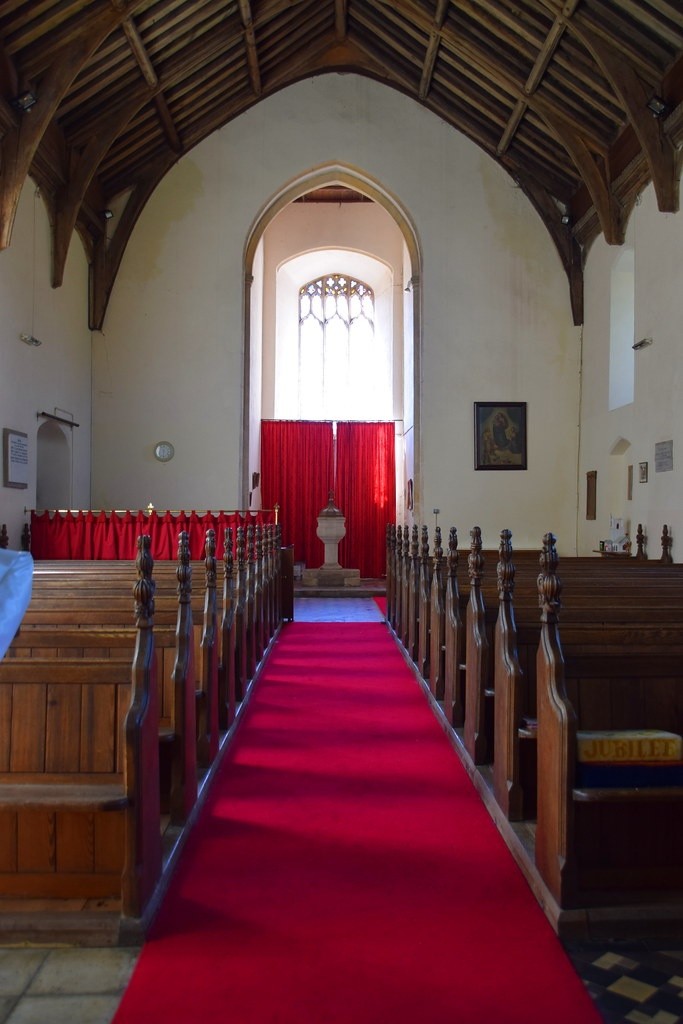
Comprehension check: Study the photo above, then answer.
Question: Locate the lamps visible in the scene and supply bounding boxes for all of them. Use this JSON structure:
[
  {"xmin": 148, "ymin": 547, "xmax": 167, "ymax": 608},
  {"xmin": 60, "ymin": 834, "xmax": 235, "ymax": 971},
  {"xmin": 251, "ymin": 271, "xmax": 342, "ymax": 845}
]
[
  {"xmin": 632, "ymin": 339, "xmax": 653, "ymax": 350},
  {"xmin": 20, "ymin": 332, "xmax": 43, "ymax": 348},
  {"xmin": 100, "ymin": 208, "xmax": 114, "ymax": 220},
  {"xmin": 11, "ymin": 88, "xmax": 38, "ymax": 108},
  {"xmin": 648, "ymin": 94, "xmax": 668, "ymax": 116},
  {"xmin": 560, "ymin": 212, "xmax": 574, "ymax": 227}
]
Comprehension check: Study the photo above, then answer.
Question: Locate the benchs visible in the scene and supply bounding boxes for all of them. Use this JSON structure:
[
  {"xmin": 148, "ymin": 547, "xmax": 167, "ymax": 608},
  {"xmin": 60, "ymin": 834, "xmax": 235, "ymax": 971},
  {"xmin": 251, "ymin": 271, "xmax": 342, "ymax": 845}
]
[
  {"xmin": 384, "ymin": 514, "xmax": 683, "ymax": 929},
  {"xmin": 0, "ymin": 522, "xmax": 289, "ymax": 940}
]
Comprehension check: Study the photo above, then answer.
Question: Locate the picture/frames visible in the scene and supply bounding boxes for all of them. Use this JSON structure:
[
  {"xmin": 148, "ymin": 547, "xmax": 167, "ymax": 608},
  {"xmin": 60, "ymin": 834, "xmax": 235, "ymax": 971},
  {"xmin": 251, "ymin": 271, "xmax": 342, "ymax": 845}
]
[{"xmin": 640, "ymin": 461, "xmax": 648, "ymax": 483}]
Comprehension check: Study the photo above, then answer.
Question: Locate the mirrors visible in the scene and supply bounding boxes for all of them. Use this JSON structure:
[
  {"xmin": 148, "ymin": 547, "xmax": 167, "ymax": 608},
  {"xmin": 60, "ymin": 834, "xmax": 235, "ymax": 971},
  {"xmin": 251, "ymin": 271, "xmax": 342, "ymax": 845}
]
[{"xmin": 474, "ymin": 401, "xmax": 527, "ymax": 471}]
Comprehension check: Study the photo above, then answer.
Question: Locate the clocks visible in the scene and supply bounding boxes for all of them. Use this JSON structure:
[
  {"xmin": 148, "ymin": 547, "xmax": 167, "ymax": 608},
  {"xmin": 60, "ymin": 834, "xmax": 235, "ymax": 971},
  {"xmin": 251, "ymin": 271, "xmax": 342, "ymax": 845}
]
[{"xmin": 155, "ymin": 441, "xmax": 174, "ymax": 462}]
